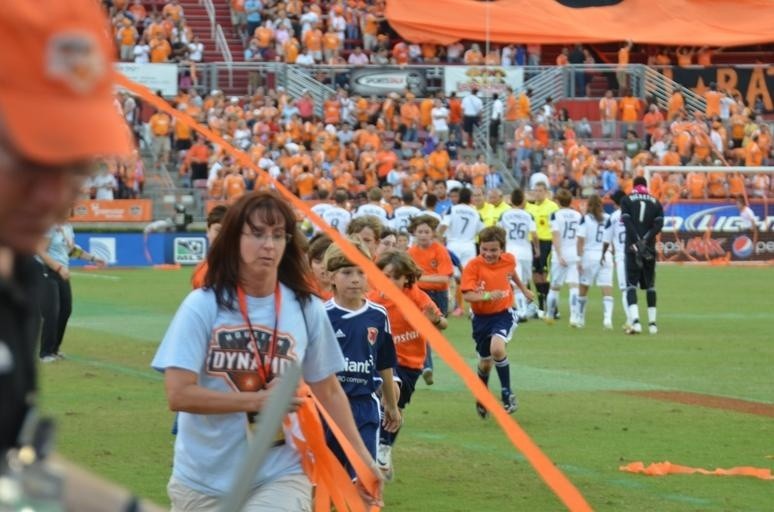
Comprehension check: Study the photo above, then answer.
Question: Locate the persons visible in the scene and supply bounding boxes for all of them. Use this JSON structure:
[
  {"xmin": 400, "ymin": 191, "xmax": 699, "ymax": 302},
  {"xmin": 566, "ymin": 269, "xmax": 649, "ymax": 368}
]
[
  {"xmin": 0, "ymin": 0, "xmax": 170, "ymax": 512},
  {"xmin": 33, "ymin": 222, "xmax": 106, "ymax": 362}
]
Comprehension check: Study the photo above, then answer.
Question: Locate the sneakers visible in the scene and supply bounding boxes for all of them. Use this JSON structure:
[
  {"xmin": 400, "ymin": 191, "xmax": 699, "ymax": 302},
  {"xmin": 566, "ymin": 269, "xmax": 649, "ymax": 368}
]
[
  {"xmin": 452, "ymin": 307, "xmax": 463, "ymax": 317},
  {"xmin": 376, "ymin": 441, "xmax": 392, "ymax": 471},
  {"xmin": 516, "ymin": 310, "xmax": 561, "ymax": 324},
  {"xmin": 501, "ymin": 391, "xmax": 519, "ymax": 415},
  {"xmin": 422, "ymin": 368, "xmax": 434, "ymax": 385},
  {"xmin": 476, "ymin": 400, "xmax": 488, "ymax": 418},
  {"xmin": 570, "ymin": 316, "xmax": 659, "ymax": 335}
]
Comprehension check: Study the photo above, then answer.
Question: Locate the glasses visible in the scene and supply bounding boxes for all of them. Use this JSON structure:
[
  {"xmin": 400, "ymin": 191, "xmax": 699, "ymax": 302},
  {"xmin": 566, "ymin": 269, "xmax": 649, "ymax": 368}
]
[{"xmin": 242, "ymin": 230, "xmax": 293, "ymax": 243}]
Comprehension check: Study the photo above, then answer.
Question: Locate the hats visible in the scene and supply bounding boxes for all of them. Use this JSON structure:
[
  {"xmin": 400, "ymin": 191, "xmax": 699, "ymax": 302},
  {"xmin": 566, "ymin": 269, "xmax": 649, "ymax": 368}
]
[{"xmin": 0, "ymin": 0, "xmax": 139, "ymax": 168}]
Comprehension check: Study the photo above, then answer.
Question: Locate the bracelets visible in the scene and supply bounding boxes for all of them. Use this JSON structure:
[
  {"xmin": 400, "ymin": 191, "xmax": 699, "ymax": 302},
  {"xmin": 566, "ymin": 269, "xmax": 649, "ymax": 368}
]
[{"xmin": 57, "ymin": 266, "xmax": 62, "ymax": 273}]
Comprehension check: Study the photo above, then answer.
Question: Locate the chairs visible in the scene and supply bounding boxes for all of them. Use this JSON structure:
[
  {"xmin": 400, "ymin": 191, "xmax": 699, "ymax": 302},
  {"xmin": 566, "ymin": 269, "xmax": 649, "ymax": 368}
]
[
  {"xmin": 501, "ymin": 117, "xmax": 642, "ymax": 177},
  {"xmin": 126, "ymin": 0, "xmax": 198, "ymax": 122}
]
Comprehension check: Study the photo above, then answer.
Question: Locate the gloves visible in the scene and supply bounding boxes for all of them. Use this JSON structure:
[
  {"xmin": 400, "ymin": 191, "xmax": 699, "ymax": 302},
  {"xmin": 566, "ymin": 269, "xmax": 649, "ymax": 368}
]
[{"xmin": 628, "ymin": 240, "xmax": 656, "ymax": 261}]
